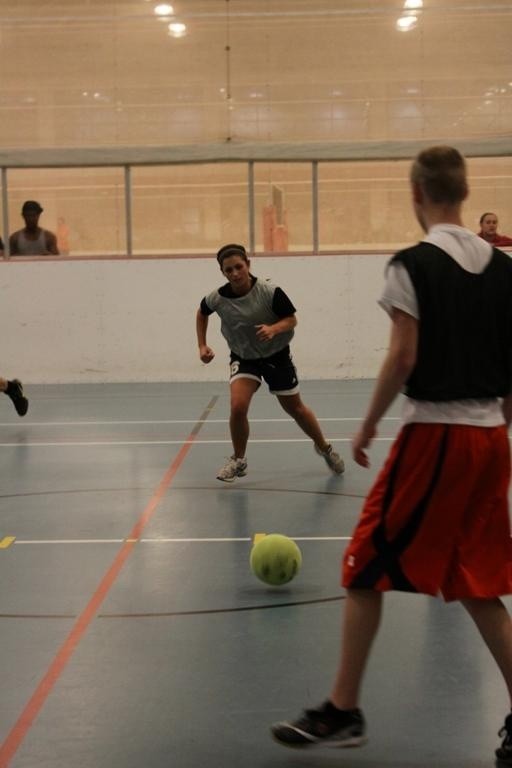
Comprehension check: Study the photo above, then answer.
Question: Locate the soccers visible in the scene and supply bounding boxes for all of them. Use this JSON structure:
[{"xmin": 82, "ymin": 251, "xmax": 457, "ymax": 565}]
[{"xmin": 251, "ymin": 534, "xmax": 302, "ymax": 583}]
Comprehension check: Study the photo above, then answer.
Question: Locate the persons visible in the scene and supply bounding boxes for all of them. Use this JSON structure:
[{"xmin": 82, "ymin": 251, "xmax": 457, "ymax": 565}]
[
  {"xmin": 197, "ymin": 245, "xmax": 345, "ymax": 482},
  {"xmin": 476, "ymin": 213, "xmax": 512, "ymax": 247},
  {"xmin": 0, "ymin": 201, "xmax": 70, "ymax": 256},
  {"xmin": 0, "ymin": 376, "xmax": 28, "ymax": 416},
  {"xmin": 270, "ymin": 145, "xmax": 512, "ymax": 768}
]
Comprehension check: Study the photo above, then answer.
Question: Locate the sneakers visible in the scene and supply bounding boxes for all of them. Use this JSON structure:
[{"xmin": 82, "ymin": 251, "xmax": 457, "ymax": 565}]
[
  {"xmin": 8, "ymin": 379, "xmax": 29, "ymax": 416},
  {"xmin": 271, "ymin": 698, "xmax": 368, "ymax": 749},
  {"xmin": 314, "ymin": 442, "xmax": 345, "ymax": 474},
  {"xmin": 494, "ymin": 712, "xmax": 511, "ymax": 768},
  {"xmin": 216, "ymin": 455, "xmax": 248, "ymax": 482}
]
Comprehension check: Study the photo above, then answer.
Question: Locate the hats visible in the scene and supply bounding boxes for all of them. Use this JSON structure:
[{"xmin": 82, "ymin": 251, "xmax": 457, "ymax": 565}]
[{"xmin": 22, "ymin": 200, "xmax": 42, "ymax": 215}]
